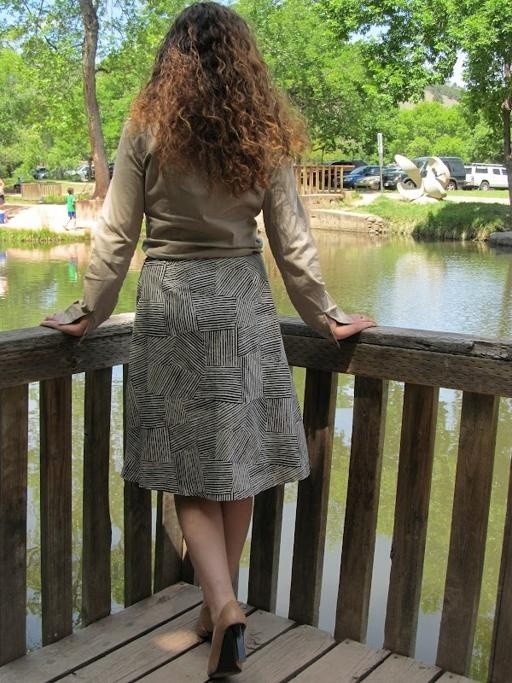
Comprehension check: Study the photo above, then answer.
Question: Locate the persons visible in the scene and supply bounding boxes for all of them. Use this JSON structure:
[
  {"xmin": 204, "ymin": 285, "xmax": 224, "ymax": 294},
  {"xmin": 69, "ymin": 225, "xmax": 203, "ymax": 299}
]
[
  {"xmin": 41, "ymin": 3, "xmax": 376, "ymax": 675},
  {"xmin": 14, "ymin": 177, "xmax": 22, "ymax": 194},
  {"xmin": 0, "ymin": 177, "xmax": 7, "ymax": 222},
  {"xmin": 63, "ymin": 187, "xmax": 79, "ymax": 230}
]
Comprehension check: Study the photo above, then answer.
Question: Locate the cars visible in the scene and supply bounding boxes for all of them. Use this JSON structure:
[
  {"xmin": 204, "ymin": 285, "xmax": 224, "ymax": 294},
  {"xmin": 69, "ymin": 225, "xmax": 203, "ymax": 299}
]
[
  {"xmin": 32, "ymin": 162, "xmax": 115, "ymax": 183},
  {"xmin": 309, "ymin": 160, "xmax": 383, "ymax": 189}
]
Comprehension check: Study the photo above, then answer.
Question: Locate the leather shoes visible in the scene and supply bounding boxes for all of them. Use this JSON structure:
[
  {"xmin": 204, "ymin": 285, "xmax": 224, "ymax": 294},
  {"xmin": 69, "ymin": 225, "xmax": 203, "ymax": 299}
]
[{"xmin": 195, "ymin": 604, "xmax": 214, "ymax": 646}]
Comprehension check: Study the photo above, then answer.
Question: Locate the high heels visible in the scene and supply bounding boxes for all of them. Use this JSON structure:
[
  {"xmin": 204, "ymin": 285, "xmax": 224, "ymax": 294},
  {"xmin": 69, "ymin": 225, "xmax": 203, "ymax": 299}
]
[{"xmin": 207, "ymin": 602, "xmax": 246, "ymax": 682}]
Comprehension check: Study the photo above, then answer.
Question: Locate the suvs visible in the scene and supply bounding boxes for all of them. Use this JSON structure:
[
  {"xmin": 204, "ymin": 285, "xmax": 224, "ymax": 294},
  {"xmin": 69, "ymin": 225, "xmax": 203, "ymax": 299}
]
[
  {"xmin": 382, "ymin": 156, "xmax": 465, "ymax": 191},
  {"xmin": 465, "ymin": 161, "xmax": 508, "ymax": 190}
]
